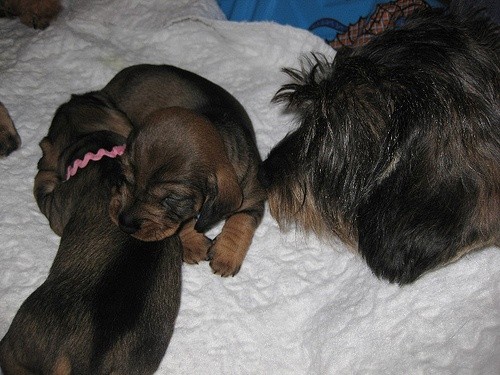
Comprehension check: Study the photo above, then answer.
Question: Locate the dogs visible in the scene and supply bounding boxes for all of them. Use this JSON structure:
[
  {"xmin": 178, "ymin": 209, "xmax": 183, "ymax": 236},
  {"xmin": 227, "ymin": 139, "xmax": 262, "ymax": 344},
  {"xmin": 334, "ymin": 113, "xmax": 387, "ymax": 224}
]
[
  {"xmin": 0, "ymin": 90, "xmax": 184, "ymax": 375},
  {"xmin": 256, "ymin": 0, "xmax": 500, "ymax": 286},
  {"xmin": 0, "ymin": 0, "xmax": 65, "ymax": 31},
  {"xmin": 43, "ymin": 64, "xmax": 268, "ymax": 278}
]
